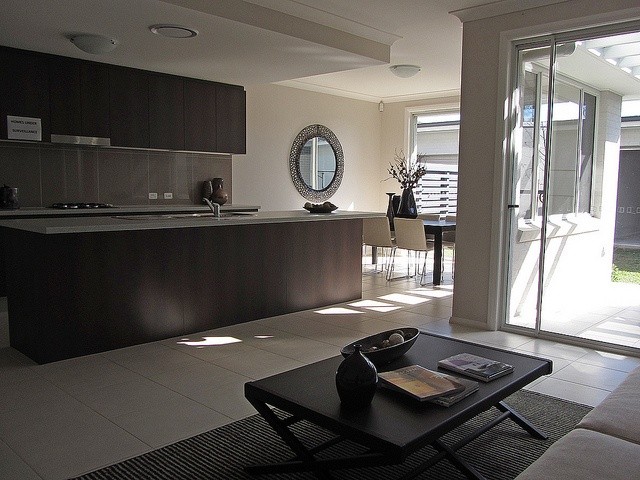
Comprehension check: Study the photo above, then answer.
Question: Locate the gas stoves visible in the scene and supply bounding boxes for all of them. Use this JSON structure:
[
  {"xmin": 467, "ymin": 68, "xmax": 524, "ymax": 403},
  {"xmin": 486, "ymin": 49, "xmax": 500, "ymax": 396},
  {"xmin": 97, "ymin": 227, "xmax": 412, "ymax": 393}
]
[{"xmin": 45, "ymin": 202, "xmax": 119, "ymax": 211}]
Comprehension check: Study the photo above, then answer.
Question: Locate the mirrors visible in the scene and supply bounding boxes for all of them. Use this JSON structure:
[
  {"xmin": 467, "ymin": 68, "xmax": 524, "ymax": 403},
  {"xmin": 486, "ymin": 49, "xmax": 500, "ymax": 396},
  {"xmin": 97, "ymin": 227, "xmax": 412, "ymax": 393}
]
[{"xmin": 289, "ymin": 124, "xmax": 345, "ymax": 204}]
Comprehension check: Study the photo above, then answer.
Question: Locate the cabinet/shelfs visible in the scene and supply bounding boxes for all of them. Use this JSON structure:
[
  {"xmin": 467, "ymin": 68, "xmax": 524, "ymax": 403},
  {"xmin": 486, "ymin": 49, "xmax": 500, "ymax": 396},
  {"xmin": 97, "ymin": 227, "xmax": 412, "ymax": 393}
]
[
  {"xmin": 149, "ymin": 70, "xmax": 185, "ymax": 151},
  {"xmin": 183, "ymin": 76, "xmax": 217, "ymax": 153},
  {"xmin": 51, "ymin": 53, "xmax": 111, "ymax": 147},
  {"xmin": 0, "ymin": 45, "xmax": 51, "ymax": 146},
  {"xmin": 215, "ymin": 81, "xmax": 246, "ymax": 154},
  {"xmin": 110, "ymin": 64, "xmax": 150, "ymax": 149}
]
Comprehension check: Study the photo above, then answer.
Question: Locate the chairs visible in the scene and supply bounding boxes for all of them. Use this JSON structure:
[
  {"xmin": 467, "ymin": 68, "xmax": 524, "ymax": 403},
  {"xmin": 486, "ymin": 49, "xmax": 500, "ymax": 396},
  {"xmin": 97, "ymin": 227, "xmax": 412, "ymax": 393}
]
[
  {"xmin": 388, "ymin": 218, "xmax": 435, "ymax": 289},
  {"xmin": 363, "ymin": 214, "xmax": 410, "ymax": 280}
]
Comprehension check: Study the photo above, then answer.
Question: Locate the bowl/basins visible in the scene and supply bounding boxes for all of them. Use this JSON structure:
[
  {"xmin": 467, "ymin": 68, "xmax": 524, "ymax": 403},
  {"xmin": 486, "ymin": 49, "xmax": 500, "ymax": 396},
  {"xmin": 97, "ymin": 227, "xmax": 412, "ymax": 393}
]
[{"xmin": 341, "ymin": 328, "xmax": 420, "ymax": 363}]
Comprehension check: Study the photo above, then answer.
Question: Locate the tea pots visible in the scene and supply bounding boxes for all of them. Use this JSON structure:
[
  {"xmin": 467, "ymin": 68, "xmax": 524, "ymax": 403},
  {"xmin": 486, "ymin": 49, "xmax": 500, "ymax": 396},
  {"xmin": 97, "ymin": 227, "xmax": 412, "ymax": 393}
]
[{"xmin": 1, "ymin": 186, "xmax": 19, "ymax": 209}]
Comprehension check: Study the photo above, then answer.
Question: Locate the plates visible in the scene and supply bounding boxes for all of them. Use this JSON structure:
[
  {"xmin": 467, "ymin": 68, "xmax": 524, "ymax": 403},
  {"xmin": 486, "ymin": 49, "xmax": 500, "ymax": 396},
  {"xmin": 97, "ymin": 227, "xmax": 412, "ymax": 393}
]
[{"xmin": 303, "ymin": 206, "xmax": 338, "ymax": 213}]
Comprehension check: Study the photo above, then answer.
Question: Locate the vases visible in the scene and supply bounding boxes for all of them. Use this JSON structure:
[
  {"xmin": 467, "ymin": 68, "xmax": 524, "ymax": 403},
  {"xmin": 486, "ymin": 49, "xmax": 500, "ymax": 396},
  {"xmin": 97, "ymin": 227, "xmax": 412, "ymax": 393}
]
[
  {"xmin": 386, "ymin": 193, "xmax": 395, "ymax": 231},
  {"xmin": 396, "ymin": 189, "xmax": 418, "ymax": 218}
]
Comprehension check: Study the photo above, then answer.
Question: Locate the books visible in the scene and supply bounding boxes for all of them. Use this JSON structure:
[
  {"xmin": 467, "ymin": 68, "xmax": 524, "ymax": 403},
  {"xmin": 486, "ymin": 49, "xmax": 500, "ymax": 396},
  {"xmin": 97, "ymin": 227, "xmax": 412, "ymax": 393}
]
[
  {"xmin": 381, "ymin": 371, "xmax": 479, "ymax": 408},
  {"xmin": 438, "ymin": 352, "xmax": 515, "ymax": 382},
  {"xmin": 377, "ymin": 364, "xmax": 465, "ymax": 402}
]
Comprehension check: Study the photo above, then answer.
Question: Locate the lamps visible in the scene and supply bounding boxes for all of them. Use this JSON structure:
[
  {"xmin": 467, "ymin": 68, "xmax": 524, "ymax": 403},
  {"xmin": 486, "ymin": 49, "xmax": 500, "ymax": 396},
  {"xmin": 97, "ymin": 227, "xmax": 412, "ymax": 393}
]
[
  {"xmin": 390, "ymin": 64, "xmax": 421, "ymax": 80},
  {"xmin": 68, "ymin": 33, "xmax": 119, "ymax": 56}
]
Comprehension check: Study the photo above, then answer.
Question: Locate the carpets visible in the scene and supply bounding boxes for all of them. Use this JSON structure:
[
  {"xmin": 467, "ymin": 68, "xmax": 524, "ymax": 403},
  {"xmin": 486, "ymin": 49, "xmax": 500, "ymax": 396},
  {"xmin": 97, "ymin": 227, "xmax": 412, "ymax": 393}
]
[{"xmin": 69, "ymin": 387, "xmax": 594, "ymax": 480}]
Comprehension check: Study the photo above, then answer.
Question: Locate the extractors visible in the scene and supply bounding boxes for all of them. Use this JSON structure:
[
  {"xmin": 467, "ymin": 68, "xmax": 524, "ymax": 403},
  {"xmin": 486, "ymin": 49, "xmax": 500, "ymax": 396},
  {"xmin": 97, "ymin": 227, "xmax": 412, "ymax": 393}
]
[{"xmin": 50, "ymin": 134, "xmax": 110, "ymax": 150}]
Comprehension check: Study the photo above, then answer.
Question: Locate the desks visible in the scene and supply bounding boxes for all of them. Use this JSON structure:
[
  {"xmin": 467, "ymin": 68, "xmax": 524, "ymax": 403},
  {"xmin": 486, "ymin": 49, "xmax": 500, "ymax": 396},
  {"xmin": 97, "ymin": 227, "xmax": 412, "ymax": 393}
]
[
  {"xmin": 244, "ymin": 330, "xmax": 552, "ymax": 479},
  {"xmin": 371, "ymin": 217, "xmax": 456, "ymax": 287}
]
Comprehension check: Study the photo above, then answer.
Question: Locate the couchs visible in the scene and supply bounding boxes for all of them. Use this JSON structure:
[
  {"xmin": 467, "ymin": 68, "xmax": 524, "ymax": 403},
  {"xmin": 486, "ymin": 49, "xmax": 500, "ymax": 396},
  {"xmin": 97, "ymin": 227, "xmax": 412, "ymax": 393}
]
[{"xmin": 515, "ymin": 364, "xmax": 640, "ymax": 480}]
